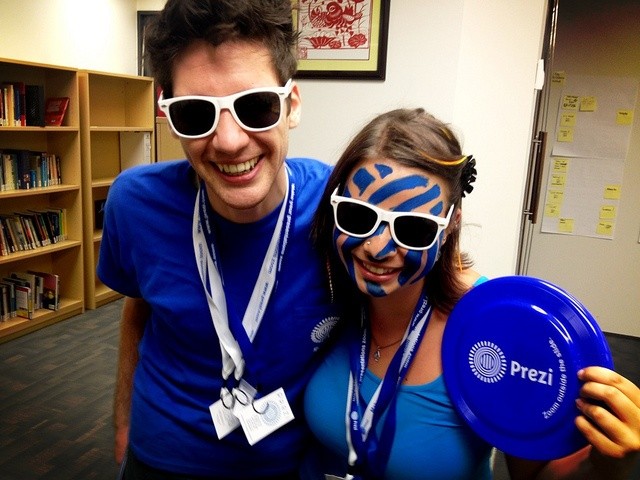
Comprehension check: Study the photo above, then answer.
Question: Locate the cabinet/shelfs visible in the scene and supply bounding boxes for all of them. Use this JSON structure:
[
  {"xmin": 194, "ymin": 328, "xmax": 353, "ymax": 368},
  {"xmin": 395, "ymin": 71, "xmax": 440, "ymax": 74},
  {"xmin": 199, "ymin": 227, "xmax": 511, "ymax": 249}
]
[
  {"xmin": 78, "ymin": 68, "xmax": 155, "ymax": 311},
  {"xmin": 0, "ymin": 58, "xmax": 86, "ymax": 344}
]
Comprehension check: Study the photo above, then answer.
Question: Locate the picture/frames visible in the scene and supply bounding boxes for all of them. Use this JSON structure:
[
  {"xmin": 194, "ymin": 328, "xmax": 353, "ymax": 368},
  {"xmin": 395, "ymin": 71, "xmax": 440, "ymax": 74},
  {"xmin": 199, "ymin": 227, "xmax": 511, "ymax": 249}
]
[{"xmin": 288, "ymin": 0, "xmax": 389, "ymax": 81}]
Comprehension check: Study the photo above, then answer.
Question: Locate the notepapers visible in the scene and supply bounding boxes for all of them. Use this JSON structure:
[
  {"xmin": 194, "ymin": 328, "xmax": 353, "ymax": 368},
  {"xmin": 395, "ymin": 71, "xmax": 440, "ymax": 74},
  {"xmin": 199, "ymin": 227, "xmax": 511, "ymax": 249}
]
[
  {"xmin": 581, "ymin": 96, "xmax": 597, "ymax": 110},
  {"xmin": 616, "ymin": 110, "xmax": 633, "ymax": 125},
  {"xmin": 604, "ymin": 185, "xmax": 621, "ymax": 199},
  {"xmin": 549, "ymin": 190, "xmax": 564, "ymax": 204},
  {"xmin": 545, "ymin": 203, "xmax": 560, "ymax": 217},
  {"xmin": 600, "ymin": 204, "xmax": 616, "ymax": 218},
  {"xmin": 559, "ymin": 217, "xmax": 574, "ymax": 233},
  {"xmin": 560, "ymin": 113, "xmax": 576, "ymax": 126},
  {"xmin": 552, "ymin": 173, "xmax": 566, "ymax": 186},
  {"xmin": 553, "ymin": 159, "xmax": 568, "ymax": 171},
  {"xmin": 552, "ymin": 71, "xmax": 566, "ymax": 87},
  {"xmin": 558, "ymin": 128, "xmax": 573, "ymax": 142},
  {"xmin": 562, "ymin": 94, "xmax": 579, "ymax": 110},
  {"xmin": 597, "ymin": 221, "xmax": 614, "ymax": 234}
]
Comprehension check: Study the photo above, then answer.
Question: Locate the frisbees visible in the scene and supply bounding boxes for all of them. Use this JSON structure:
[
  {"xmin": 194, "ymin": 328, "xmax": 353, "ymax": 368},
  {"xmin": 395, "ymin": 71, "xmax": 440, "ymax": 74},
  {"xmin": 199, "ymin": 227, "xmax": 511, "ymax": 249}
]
[{"xmin": 440, "ymin": 275, "xmax": 614, "ymax": 462}]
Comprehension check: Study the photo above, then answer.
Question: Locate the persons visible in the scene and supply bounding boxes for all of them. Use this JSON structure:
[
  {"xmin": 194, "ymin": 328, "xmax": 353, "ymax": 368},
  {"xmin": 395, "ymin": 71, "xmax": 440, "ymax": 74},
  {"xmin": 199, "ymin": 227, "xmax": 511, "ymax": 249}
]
[
  {"xmin": 97, "ymin": 0, "xmax": 503, "ymax": 479},
  {"xmin": 304, "ymin": 108, "xmax": 640, "ymax": 480}
]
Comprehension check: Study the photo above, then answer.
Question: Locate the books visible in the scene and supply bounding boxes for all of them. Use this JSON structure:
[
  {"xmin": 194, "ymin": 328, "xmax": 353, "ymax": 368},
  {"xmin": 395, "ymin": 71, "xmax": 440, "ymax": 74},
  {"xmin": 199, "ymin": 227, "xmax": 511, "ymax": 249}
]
[
  {"xmin": 120, "ymin": 131, "xmax": 151, "ymax": 173},
  {"xmin": 0, "ymin": 207, "xmax": 68, "ymax": 256},
  {"xmin": 95, "ymin": 198, "xmax": 107, "ymax": 229},
  {"xmin": 26, "ymin": 85, "xmax": 45, "ymax": 126},
  {"xmin": 0, "ymin": 152, "xmax": 62, "ymax": 191},
  {"xmin": 0, "ymin": 81, "xmax": 26, "ymax": 126},
  {"xmin": 0, "ymin": 270, "xmax": 59, "ymax": 322},
  {"xmin": 43, "ymin": 97, "xmax": 71, "ymax": 126}
]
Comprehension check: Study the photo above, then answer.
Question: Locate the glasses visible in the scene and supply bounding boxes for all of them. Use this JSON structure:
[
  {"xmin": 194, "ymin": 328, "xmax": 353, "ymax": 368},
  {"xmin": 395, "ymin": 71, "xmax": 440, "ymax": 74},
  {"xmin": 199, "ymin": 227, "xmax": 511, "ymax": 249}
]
[
  {"xmin": 330, "ymin": 184, "xmax": 455, "ymax": 250},
  {"xmin": 157, "ymin": 78, "xmax": 292, "ymax": 138}
]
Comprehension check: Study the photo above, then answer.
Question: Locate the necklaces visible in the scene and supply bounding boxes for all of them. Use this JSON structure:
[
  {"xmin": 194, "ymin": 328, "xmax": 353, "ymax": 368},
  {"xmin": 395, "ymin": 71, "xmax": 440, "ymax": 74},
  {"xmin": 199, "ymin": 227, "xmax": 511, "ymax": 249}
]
[{"xmin": 371, "ymin": 333, "xmax": 403, "ymax": 361}]
